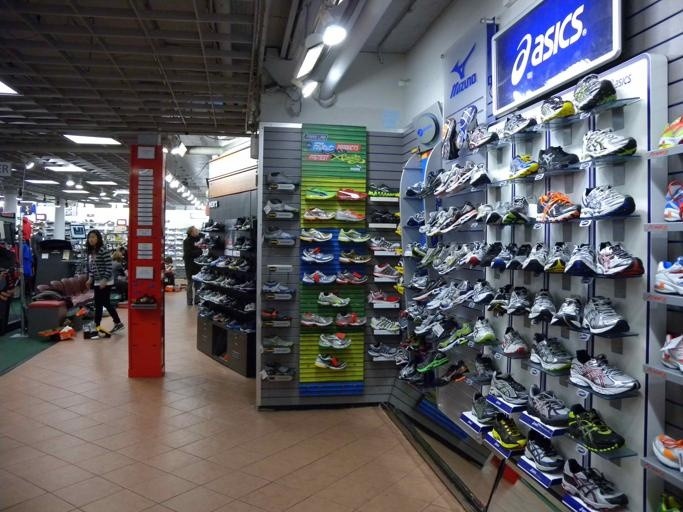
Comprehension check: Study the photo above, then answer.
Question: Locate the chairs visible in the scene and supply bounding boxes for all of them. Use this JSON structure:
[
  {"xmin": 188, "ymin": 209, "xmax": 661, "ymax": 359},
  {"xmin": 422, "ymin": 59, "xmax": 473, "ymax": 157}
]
[{"xmin": 33, "ymin": 273, "xmax": 104, "ymax": 307}]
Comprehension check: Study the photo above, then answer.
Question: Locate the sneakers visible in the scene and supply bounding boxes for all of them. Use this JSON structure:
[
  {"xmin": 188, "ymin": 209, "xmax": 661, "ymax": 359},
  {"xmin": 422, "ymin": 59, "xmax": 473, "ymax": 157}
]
[
  {"xmin": 258, "ymin": 170, "xmax": 408, "ymax": 381},
  {"xmin": 401, "ymin": 71, "xmax": 642, "ymax": 511},
  {"xmin": 90, "ymin": 320, "xmax": 123, "ymax": 334},
  {"xmin": 134, "ymin": 295, "xmax": 156, "ymax": 305},
  {"xmin": 655, "ymin": 116, "xmax": 683, "ymax": 511},
  {"xmin": 191, "ymin": 215, "xmax": 254, "ymax": 333}
]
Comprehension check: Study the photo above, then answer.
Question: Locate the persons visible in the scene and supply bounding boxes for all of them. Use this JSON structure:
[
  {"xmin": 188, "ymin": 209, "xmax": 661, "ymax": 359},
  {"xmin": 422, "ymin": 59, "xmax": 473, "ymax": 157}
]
[
  {"xmin": 161, "ymin": 256, "xmax": 176, "ymax": 293},
  {"xmin": 182, "ymin": 225, "xmax": 205, "ymax": 306},
  {"xmin": 0, "ymin": 228, "xmax": 128, "ymax": 340}
]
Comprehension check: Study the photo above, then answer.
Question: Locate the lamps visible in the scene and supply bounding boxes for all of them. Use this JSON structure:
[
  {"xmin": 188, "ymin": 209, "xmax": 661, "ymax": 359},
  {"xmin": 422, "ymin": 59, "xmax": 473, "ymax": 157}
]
[
  {"xmin": 317, "ymin": 1, "xmax": 349, "ymax": 46},
  {"xmin": 75, "ymin": 176, "xmax": 85, "ymax": 189},
  {"xmin": 288, "ymin": 72, "xmax": 319, "ymax": 101},
  {"xmin": 292, "ymin": 32, "xmax": 325, "ymax": 81},
  {"xmin": 175, "ymin": 136, "xmax": 188, "ymax": 159},
  {"xmin": 20, "ymin": 156, "xmax": 35, "ymax": 169},
  {"xmin": 161, "ymin": 136, "xmax": 180, "ymax": 155},
  {"xmin": 64, "ymin": 174, "xmax": 74, "ymax": 187}
]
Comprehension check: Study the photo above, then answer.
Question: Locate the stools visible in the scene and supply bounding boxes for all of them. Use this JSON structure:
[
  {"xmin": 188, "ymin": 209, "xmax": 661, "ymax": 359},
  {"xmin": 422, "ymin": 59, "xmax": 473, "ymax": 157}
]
[{"xmin": 24, "ymin": 298, "xmax": 68, "ymax": 335}]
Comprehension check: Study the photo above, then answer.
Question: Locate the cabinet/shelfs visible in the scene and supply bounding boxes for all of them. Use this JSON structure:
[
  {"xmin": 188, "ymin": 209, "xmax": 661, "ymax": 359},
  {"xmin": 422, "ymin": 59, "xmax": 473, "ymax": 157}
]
[
  {"xmin": 37, "ymin": 219, "xmax": 189, "ymax": 282},
  {"xmin": 191, "ymin": 213, "xmax": 257, "ymax": 380},
  {"xmin": 403, "ymin": 93, "xmax": 683, "ymax": 507},
  {"xmin": 257, "ymin": 115, "xmax": 403, "ymax": 408}
]
